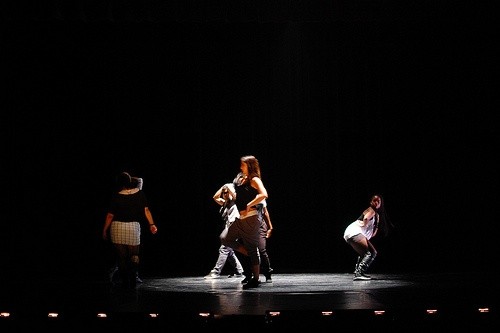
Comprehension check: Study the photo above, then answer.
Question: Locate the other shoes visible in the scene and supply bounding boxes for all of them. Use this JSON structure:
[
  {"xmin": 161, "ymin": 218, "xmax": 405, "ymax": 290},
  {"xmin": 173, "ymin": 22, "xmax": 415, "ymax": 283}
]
[
  {"xmin": 135, "ymin": 274, "xmax": 142, "ymax": 283},
  {"xmin": 204, "ymin": 272, "xmax": 220, "ymax": 279},
  {"xmin": 242, "ymin": 276, "xmax": 253, "ymax": 284},
  {"xmin": 267, "ymin": 276, "xmax": 271, "ymax": 281},
  {"xmin": 353, "ymin": 273, "xmax": 373, "ymax": 281},
  {"xmin": 243, "ymin": 278, "xmax": 259, "ymax": 289}
]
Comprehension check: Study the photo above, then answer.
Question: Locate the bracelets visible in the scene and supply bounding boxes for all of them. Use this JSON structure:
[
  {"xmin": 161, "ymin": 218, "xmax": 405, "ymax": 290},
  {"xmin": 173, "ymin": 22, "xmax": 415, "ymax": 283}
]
[{"xmin": 150, "ymin": 224, "xmax": 154, "ymax": 226}]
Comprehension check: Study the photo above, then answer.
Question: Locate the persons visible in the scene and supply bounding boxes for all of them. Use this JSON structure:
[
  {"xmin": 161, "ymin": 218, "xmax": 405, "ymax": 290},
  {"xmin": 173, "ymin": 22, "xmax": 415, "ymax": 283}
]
[
  {"xmin": 102, "ymin": 173, "xmax": 272, "ymax": 284},
  {"xmin": 220, "ymin": 156, "xmax": 268, "ymax": 287},
  {"xmin": 343, "ymin": 195, "xmax": 386, "ymax": 281}
]
